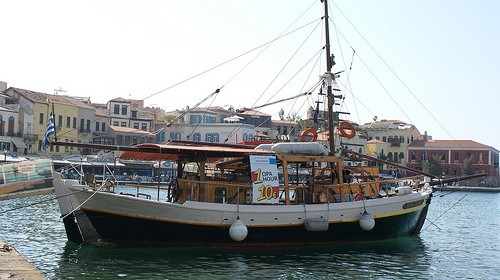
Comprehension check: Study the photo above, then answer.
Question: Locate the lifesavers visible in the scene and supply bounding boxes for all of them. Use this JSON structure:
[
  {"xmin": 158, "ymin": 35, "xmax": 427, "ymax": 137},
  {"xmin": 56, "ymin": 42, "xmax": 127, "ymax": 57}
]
[
  {"xmin": 322, "ymin": 167, "xmax": 336, "ymax": 182},
  {"xmin": 340, "ymin": 121, "xmax": 356, "ymax": 138},
  {"xmin": 301, "ymin": 128, "xmax": 317, "ymax": 142},
  {"xmin": 354, "ymin": 193, "xmax": 363, "ymax": 201}
]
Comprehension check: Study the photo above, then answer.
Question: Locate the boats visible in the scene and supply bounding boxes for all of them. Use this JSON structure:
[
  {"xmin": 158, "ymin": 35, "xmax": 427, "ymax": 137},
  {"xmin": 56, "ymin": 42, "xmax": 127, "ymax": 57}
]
[
  {"xmin": 49, "ymin": 0, "xmax": 488, "ymax": 244},
  {"xmin": 1, "ymin": 153, "xmax": 32, "ymax": 172}
]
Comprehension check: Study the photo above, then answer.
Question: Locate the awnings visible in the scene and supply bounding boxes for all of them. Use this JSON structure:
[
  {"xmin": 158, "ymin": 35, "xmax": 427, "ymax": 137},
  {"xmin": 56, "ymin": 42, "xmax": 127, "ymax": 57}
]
[{"xmin": 13, "ymin": 141, "xmax": 27, "ymax": 148}]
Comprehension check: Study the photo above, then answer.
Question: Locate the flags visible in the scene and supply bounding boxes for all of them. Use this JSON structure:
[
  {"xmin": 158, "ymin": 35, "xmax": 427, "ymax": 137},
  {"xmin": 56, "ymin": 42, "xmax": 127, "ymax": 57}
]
[{"xmin": 41, "ymin": 111, "xmax": 55, "ymax": 152}]
